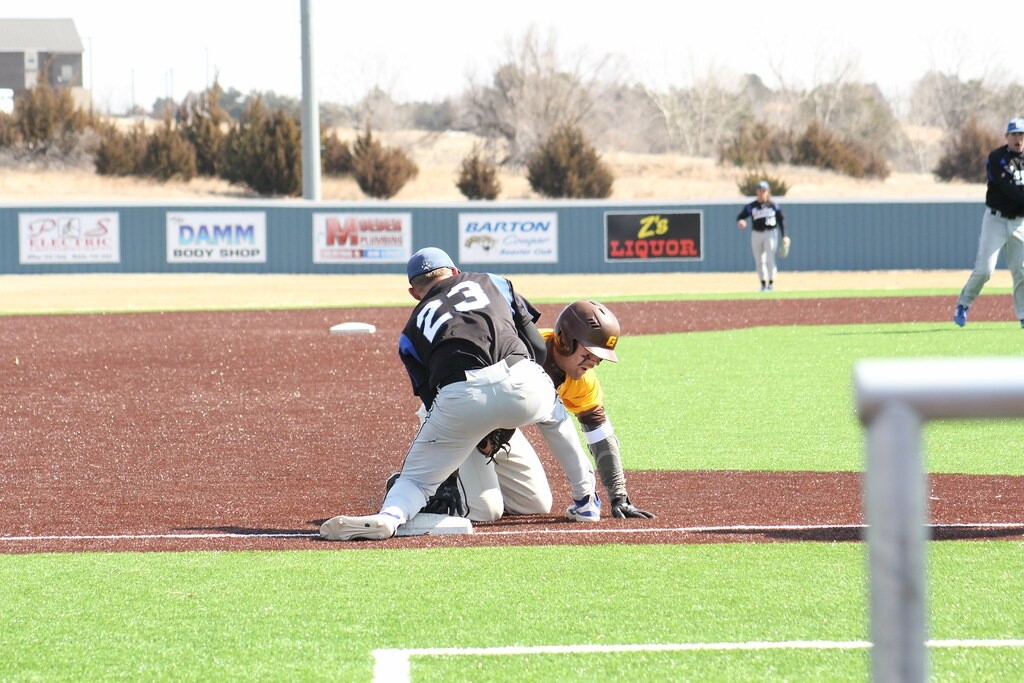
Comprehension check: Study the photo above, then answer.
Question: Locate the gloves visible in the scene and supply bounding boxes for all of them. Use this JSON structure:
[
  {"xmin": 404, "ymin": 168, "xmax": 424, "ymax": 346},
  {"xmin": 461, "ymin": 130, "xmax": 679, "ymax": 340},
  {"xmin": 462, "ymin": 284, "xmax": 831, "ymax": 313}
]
[{"xmin": 610, "ymin": 495, "xmax": 658, "ymax": 520}]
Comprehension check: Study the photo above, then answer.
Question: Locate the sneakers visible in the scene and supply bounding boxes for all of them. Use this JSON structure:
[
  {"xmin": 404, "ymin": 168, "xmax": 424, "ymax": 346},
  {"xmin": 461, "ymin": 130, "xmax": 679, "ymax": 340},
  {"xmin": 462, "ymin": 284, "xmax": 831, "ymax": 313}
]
[
  {"xmin": 953, "ymin": 302, "xmax": 969, "ymax": 327},
  {"xmin": 566, "ymin": 491, "xmax": 601, "ymax": 522},
  {"xmin": 318, "ymin": 509, "xmax": 406, "ymax": 541}
]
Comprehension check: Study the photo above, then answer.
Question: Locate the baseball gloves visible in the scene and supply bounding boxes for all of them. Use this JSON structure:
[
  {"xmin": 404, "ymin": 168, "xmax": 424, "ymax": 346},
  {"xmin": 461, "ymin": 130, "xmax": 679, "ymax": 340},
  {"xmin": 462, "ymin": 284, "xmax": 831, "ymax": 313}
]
[{"xmin": 475, "ymin": 427, "xmax": 517, "ymax": 466}]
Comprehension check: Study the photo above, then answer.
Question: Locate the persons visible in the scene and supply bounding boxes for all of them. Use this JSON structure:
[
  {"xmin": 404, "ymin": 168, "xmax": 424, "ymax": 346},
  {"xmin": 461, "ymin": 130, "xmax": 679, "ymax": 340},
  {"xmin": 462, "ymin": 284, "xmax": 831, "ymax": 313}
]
[
  {"xmin": 951, "ymin": 117, "xmax": 1024, "ymax": 327},
  {"xmin": 380, "ymin": 298, "xmax": 658, "ymax": 522},
  {"xmin": 736, "ymin": 182, "xmax": 790, "ymax": 290},
  {"xmin": 316, "ymin": 244, "xmax": 606, "ymax": 544}
]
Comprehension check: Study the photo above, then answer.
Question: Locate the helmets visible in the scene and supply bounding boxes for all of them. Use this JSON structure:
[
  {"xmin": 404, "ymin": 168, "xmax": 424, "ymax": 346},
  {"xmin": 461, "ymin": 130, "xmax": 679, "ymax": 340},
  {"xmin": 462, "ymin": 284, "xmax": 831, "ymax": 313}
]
[{"xmin": 553, "ymin": 300, "xmax": 621, "ymax": 363}]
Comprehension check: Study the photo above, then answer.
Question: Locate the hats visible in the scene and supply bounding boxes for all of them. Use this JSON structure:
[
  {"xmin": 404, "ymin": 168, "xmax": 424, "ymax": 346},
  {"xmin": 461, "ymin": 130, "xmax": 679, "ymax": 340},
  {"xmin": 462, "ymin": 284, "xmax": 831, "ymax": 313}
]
[
  {"xmin": 1008, "ymin": 118, "xmax": 1024, "ymax": 133},
  {"xmin": 406, "ymin": 246, "xmax": 461, "ymax": 284},
  {"xmin": 756, "ymin": 181, "xmax": 769, "ymax": 190}
]
